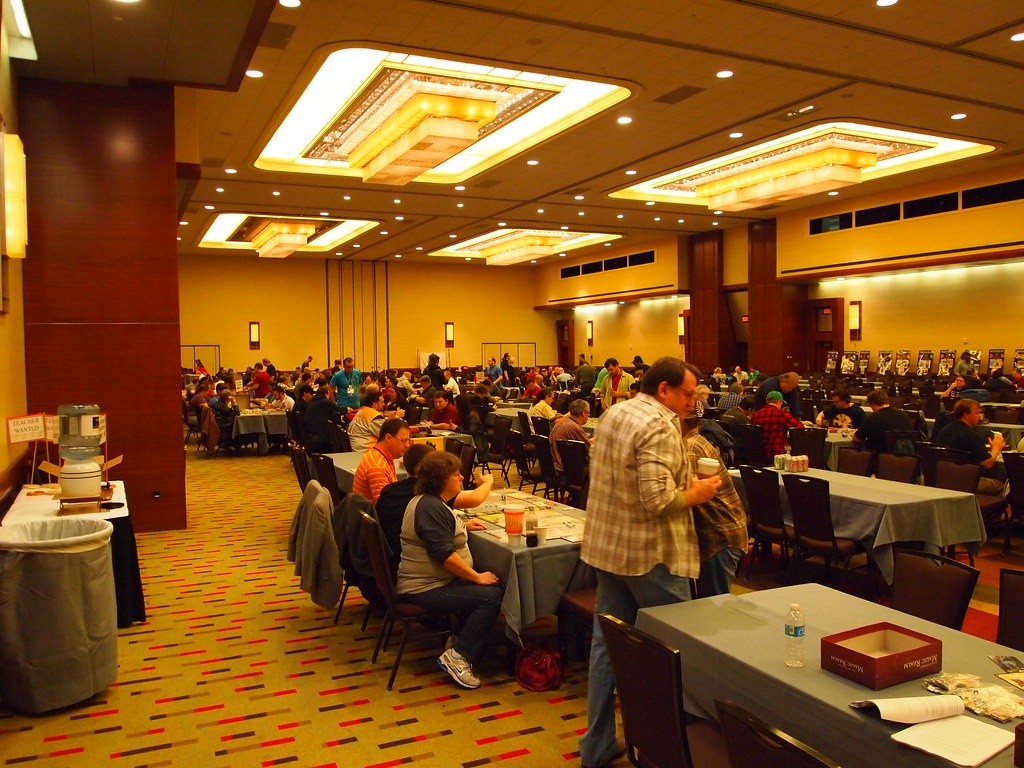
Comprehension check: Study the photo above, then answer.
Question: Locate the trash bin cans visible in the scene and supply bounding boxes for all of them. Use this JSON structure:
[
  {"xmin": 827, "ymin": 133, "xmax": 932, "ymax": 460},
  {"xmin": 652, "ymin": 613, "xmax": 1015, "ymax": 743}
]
[{"xmin": 0, "ymin": 518, "xmax": 117, "ymax": 716}]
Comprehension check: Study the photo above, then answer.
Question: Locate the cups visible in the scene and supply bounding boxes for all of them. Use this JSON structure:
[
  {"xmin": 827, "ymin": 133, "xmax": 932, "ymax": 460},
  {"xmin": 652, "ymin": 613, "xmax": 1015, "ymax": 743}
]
[
  {"xmin": 508, "ymin": 533, "xmax": 522, "ymax": 547},
  {"xmin": 533, "ymin": 526, "xmax": 548, "ymax": 546},
  {"xmin": 697, "ymin": 458, "xmax": 721, "ymax": 479}
]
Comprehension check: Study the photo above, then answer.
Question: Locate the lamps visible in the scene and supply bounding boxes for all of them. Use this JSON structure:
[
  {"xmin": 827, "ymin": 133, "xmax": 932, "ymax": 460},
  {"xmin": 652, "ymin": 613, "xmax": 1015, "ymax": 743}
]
[
  {"xmin": 850, "ymin": 305, "xmax": 860, "ymax": 330},
  {"xmin": 251, "ymin": 324, "xmax": 259, "ymax": 342},
  {"xmin": 586, "ymin": 323, "xmax": 592, "ymax": 339},
  {"xmin": 677, "ymin": 316, "xmax": 685, "ymax": 336},
  {"xmin": 446, "ymin": 324, "xmax": 453, "ymax": 340},
  {"xmin": 0, "ymin": 132, "xmax": 29, "ymax": 258},
  {"xmin": 193, "ymin": 40, "xmax": 1006, "ymax": 274}
]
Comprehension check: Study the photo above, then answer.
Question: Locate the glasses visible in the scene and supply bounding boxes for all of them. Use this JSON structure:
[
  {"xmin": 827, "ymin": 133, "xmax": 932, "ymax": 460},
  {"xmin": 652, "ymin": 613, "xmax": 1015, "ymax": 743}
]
[
  {"xmin": 672, "ymin": 384, "xmax": 695, "ymax": 399},
  {"xmin": 392, "ymin": 435, "xmax": 411, "ymax": 445}
]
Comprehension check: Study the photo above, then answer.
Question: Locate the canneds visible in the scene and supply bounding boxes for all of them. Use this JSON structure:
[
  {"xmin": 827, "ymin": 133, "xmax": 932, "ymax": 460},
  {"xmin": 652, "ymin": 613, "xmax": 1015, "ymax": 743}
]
[{"xmin": 774, "ymin": 454, "xmax": 809, "ymax": 473}]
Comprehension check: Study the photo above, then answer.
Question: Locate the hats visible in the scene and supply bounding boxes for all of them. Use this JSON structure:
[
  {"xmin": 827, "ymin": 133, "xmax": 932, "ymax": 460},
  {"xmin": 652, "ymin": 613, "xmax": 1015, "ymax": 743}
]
[
  {"xmin": 221, "ymin": 389, "xmax": 236, "ymax": 397},
  {"xmin": 767, "ymin": 391, "xmax": 787, "ymax": 404},
  {"xmin": 418, "ymin": 375, "xmax": 430, "ymax": 382},
  {"xmin": 299, "ymin": 385, "xmax": 318, "ymax": 394}
]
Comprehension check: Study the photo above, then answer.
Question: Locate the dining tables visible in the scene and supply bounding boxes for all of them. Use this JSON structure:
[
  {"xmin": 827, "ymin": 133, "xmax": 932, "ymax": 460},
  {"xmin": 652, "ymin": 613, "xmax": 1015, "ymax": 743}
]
[
  {"xmin": 450, "ymin": 489, "xmax": 588, "ymax": 672},
  {"xmin": 404, "ymin": 427, "xmax": 479, "ymax": 471},
  {"xmin": 925, "ymin": 417, "xmax": 1024, "ymax": 450},
  {"xmin": 486, "ymin": 407, "xmax": 530, "ymax": 431},
  {"xmin": 980, "ymin": 402, "xmax": 1024, "ymax": 410},
  {"xmin": 724, "ymin": 467, "xmax": 988, "ymax": 596},
  {"xmin": 2, "ymin": 481, "xmax": 146, "ymax": 629},
  {"xmin": 318, "ymin": 452, "xmax": 410, "ymax": 494},
  {"xmin": 581, "ymin": 417, "xmax": 599, "ymax": 438},
  {"xmin": 231, "ymin": 408, "xmax": 290, "ymax": 454},
  {"xmin": 497, "ymin": 403, "xmax": 533, "ymax": 409},
  {"xmin": 786, "ymin": 425, "xmax": 858, "ymax": 472},
  {"xmin": 634, "ymin": 583, "xmax": 1022, "ymax": 767}
]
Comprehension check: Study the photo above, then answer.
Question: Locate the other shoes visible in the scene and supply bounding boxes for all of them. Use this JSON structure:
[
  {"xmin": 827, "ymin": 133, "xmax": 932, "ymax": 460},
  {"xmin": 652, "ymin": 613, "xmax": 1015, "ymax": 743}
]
[{"xmin": 614, "ymin": 735, "xmax": 626, "ymax": 759}]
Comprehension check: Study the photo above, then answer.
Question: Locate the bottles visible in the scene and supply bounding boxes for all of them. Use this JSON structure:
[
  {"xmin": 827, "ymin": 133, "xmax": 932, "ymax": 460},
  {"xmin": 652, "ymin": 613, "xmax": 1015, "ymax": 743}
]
[
  {"xmin": 58, "ymin": 403, "xmax": 102, "ymax": 462},
  {"xmin": 784, "ymin": 603, "xmax": 807, "ymax": 667},
  {"xmin": 501, "ymin": 491, "xmax": 506, "ymax": 509},
  {"xmin": 526, "ymin": 506, "xmax": 538, "ymax": 547}
]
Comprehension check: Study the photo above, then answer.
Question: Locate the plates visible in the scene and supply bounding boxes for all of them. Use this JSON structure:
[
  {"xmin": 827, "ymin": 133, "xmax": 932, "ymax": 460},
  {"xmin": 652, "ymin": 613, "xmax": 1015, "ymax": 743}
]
[
  {"xmin": 802, "ymin": 420, "xmax": 857, "ymax": 438},
  {"xmin": 241, "ymin": 408, "xmax": 285, "ymax": 415}
]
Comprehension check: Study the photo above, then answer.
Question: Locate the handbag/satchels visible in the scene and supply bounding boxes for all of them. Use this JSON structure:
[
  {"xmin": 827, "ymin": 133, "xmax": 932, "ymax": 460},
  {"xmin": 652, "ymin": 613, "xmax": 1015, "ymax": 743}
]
[{"xmin": 515, "ymin": 640, "xmax": 561, "ymax": 691}]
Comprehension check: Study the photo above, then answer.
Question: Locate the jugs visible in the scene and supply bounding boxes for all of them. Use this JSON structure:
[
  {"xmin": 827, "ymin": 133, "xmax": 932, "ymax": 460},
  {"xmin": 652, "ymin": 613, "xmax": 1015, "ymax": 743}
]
[{"xmin": 504, "ymin": 504, "xmax": 525, "ymax": 534}]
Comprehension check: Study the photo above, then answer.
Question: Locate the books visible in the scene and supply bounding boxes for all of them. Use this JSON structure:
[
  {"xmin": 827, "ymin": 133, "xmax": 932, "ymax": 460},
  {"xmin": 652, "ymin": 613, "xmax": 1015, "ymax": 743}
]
[{"xmin": 850, "ymin": 695, "xmax": 1015, "ymax": 768}]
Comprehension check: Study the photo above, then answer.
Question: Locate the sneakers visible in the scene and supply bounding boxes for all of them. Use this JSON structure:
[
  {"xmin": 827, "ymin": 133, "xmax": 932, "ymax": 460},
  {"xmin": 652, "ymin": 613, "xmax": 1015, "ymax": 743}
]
[
  {"xmin": 436, "ymin": 647, "xmax": 481, "ymax": 689},
  {"xmin": 446, "ymin": 635, "xmax": 458, "ymax": 650}
]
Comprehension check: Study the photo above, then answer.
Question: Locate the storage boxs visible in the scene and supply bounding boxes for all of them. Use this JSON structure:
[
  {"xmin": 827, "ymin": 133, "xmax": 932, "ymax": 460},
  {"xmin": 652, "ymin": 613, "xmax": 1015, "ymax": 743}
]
[
  {"xmin": 38, "ymin": 455, "xmax": 123, "ymax": 484},
  {"xmin": 820, "ymin": 621, "xmax": 943, "ymax": 691}
]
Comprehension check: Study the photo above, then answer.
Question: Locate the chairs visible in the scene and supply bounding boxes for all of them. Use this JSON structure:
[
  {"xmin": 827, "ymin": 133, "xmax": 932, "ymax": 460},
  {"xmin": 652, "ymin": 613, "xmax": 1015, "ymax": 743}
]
[{"xmin": 181, "ymin": 368, "xmax": 1024, "ymax": 768}]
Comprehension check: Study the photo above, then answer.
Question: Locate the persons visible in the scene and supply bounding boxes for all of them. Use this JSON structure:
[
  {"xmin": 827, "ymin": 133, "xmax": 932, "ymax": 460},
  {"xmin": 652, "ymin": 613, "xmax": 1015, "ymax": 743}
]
[
  {"xmin": 353, "ymin": 418, "xmax": 411, "ymax": 502},
  {"xmin": 580, "ymin": 356, "xmax": 722, "ymax": 768},
  {"xmin": 395, "ymin": 451, "xmax": 502, "ymax": 689},
  {"xmin": 375, "ymin": 443, "xmax": 494, "ymax": 561},
  {"xmin": 186, "ymin": 352, "xmax": 650, "ymax": 481},
  {"xmin": 682, "ymin": 352, "xmax": 1024, "ymax": 597}
]
